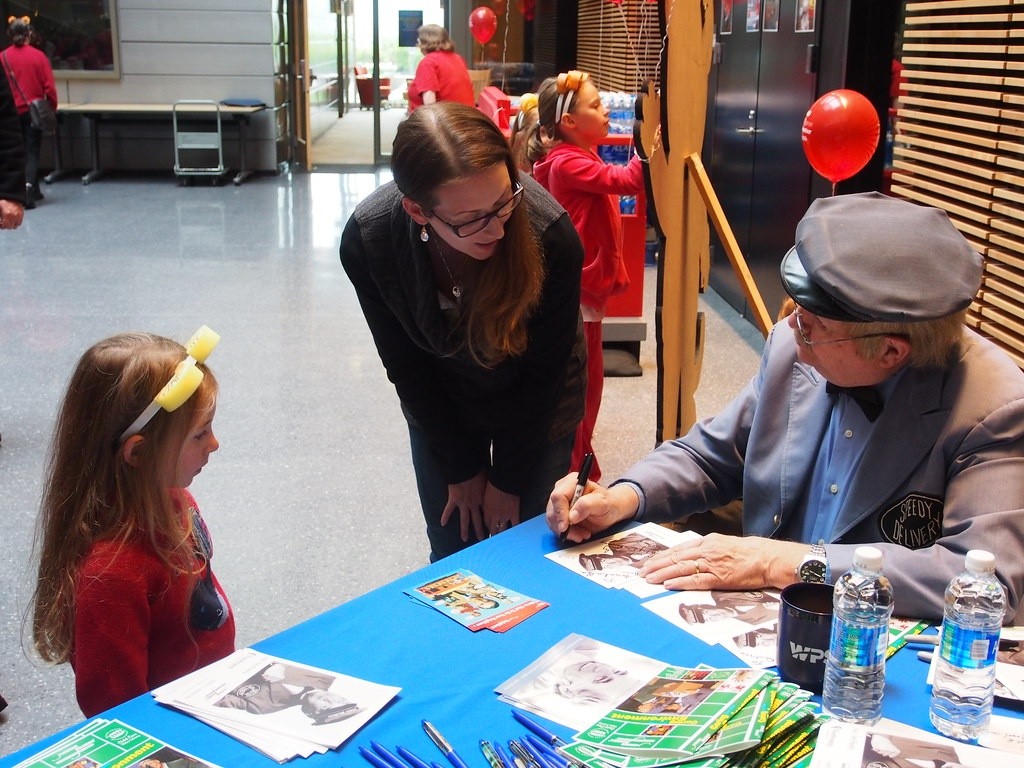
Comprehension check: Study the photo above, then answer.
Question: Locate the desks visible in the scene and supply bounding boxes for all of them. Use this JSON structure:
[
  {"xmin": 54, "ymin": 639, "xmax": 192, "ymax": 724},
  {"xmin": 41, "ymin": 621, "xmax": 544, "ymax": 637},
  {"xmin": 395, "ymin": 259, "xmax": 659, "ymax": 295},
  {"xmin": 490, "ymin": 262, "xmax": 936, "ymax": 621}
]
[
  {"xmin": 356, "ymin": 75, "xmax": 390, "ymax": 110},
  {"xmin": 42, "ymin": 102, "xmax": 277, "ymax": 185},
  {"xmin": 0, "ymin": 513, "xmax": 1024, "ymax": 768}
]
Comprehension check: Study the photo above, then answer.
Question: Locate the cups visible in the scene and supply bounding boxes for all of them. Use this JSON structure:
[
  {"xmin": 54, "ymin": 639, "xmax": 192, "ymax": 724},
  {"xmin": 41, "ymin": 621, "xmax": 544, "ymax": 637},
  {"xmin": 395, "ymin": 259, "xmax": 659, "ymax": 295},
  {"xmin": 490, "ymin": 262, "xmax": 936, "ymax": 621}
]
[{"xmin": 778, "ymin": 582, "xmax": 835, "ymax": 694}]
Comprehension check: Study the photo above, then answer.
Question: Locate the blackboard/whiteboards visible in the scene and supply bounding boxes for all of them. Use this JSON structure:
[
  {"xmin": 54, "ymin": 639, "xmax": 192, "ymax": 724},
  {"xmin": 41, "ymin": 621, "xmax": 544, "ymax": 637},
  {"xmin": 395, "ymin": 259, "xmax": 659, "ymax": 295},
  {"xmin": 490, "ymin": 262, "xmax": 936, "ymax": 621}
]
[{"xmin": 0, "ymin": 0, "xmax": 121, "ymax": 80}]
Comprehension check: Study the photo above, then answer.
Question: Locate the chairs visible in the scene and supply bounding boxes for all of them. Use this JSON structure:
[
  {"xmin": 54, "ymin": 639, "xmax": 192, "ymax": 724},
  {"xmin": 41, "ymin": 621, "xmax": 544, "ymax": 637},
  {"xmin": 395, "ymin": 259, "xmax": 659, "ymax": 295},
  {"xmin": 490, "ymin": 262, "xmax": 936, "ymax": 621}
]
[{"xmin": 467, "ymin": 69, "xmax": 492, "ymax": 106}]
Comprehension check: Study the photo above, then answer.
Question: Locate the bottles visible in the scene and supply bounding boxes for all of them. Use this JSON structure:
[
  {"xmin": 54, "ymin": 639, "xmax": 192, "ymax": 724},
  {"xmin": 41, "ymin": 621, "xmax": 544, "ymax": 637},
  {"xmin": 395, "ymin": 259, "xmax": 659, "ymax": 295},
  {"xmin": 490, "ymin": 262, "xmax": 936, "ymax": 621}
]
[
  {"xmin": 597, "ymin": 91, "xmax": 637, "ymax": 166},
  {"xmin": 617, "ymin": 193, "xmax": 636, "ymax": 215},
  {"xmin": 821, "ymin": 545, "xmax": 895, "ymax": 727},
  {"xmin": 929, "ymin": 549, "xmax": 1006, "ymax": 741}
]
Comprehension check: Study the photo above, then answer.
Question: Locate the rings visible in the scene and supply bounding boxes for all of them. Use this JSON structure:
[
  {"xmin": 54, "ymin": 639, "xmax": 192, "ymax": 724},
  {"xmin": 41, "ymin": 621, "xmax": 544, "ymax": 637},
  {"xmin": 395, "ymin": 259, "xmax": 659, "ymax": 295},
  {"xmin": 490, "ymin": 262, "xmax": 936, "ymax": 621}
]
[
  {"xmin": 692, "ymin": 558, "xmax": 700, "ymax": 575},
  {"xmin": 493, "ymin": 522, "xmax": 507, "ymax": 529}
]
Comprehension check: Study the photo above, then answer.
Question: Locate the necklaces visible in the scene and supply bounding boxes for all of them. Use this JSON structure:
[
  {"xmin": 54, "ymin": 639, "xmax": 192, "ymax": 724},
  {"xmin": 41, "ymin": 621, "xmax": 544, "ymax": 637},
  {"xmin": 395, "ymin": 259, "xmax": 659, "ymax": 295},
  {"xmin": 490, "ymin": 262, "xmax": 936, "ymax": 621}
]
[{"xmin": 429, "ymin": 226, "xmax": 470, "ymax": 298}]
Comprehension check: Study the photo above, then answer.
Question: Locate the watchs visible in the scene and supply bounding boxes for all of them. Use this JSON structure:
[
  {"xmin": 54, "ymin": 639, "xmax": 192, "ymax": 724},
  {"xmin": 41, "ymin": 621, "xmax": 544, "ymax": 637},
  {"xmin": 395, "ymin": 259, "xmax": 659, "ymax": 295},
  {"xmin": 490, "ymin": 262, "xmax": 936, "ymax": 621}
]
[{"xmin": 792, "ymin": 544, "xmax": 827, "ymax": 585}]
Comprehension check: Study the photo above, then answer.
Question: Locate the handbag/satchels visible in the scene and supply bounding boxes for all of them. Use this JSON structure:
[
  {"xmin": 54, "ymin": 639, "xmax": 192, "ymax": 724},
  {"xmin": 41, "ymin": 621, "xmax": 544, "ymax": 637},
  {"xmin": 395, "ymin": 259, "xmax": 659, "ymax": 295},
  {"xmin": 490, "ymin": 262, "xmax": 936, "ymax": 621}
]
[{"xmin": 28, "ymin": 99, "xmax": 57, "ymax": 132}]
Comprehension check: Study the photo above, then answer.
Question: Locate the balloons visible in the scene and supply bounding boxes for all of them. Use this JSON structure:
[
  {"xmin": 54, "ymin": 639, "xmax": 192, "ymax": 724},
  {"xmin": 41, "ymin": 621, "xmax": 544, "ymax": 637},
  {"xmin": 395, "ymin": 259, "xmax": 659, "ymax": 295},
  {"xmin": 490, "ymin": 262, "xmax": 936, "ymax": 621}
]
[
  {"xmin": 801, "ymin": 89, "xmax": 881, "ymax": 183},
  {"xmin": 468, "ymin": 6, "xmax": 498, "ymax": 44}
]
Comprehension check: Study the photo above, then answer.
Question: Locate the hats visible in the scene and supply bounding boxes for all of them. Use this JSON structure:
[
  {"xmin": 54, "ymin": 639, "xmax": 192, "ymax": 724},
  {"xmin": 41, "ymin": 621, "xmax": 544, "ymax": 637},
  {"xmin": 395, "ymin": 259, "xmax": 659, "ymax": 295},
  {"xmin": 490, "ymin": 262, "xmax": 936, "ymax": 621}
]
[{"xmin": 781, "ymin": 192, "xmax": 985, "ymax": 322}]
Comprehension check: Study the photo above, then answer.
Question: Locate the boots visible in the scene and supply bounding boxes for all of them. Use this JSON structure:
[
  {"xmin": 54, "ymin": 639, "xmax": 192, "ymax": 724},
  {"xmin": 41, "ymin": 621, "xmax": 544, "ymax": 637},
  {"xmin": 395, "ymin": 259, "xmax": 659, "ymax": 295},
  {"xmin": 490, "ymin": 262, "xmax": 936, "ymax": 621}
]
[{"xmin": 25, "ymin": 186, "xmax": 44, "ymax": 210}]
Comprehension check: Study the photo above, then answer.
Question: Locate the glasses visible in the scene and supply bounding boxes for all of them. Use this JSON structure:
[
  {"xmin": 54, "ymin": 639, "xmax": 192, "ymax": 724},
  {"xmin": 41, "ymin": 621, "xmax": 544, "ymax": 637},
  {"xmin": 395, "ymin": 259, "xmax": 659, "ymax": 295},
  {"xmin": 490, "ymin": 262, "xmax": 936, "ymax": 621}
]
[
  {"xmin": 429, "ymin": 180, "xmax": 524, "ymax": 239},
  {"xmin": 793, "ymin": 301, "xmax": 910, "ymax": 345}
]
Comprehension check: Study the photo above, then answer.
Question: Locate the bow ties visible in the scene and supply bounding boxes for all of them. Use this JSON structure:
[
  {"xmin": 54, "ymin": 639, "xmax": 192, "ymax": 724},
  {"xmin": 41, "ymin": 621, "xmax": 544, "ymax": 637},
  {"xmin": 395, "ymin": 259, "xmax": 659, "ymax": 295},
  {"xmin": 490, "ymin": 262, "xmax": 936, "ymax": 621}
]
[{"xmin": 826, "ymin": 381, "xmax": 884, "ymax": 423}]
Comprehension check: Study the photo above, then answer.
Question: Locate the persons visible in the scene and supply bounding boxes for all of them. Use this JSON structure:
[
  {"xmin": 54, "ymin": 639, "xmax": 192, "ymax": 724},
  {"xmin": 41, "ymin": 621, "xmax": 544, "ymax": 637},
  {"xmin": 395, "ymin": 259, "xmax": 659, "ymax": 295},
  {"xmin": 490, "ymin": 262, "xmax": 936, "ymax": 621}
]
[
  {"xmin": 0, "ymin": 13, "xmax": 58, "ymax": 209},
  {"xmin": 510, "ymin": 69, "xmax": 644, "ymax": 482},
  {"xmin": 544, "ymin": 191, "xmax": 1024, "ymax": 627},
  {"xmin": 406, "ymin": 24, "xmax": 476, "ymax": 116},
  {"xmin": 19, "ymin": 326, "xmax": 236, "ymax": 719},
  {"xmin": 1, "ymin": 60, "xmax": 28, "ymax": 232},
  {"xmin": 338, "ymin": 100, "xmax": 587, "ymax": 563},
  {"xmin": 423, "ymin": 582, "xmax": 521, "ymax": 618}
]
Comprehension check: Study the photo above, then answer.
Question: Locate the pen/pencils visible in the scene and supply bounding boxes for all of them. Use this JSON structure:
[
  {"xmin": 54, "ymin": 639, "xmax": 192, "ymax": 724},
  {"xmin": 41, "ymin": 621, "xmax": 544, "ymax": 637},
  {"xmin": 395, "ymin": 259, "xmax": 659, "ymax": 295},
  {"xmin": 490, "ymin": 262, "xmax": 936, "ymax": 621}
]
[
  {"xmin": 494, "ymin": 741, "xmax": 528, "ymax": 768},
  {"xmin": 560, "ymin": 452, "xmax": 594, "ymax": 545},
  {"xmin": 421, "ymin": 719, "xmax": 468, "ymax": 768},
  {"xmin": 904, "ymin": 634, "xmax": 939, "ymax": 643},
  {"xmin": 525, "ymin": 734, "xmax": 591, "ymax": 768},
  {"xmin": 507, "ymin": 737, "xmax": 556, "ymax": 768},
  {"xmin": 370, "ymin": 741, "xmax": 412, "ymax": 768},
  {"xmin": 358, "ymin": 746, "xmax": 396, "ymax": 768},
  {"xmin": 479, "ymin": 739, "xmax": 505, "ymax": 768},
  {"xmin": 906, "ymin": 642, "xmax": 935, "ymax": 651},
  {"xmin": 396, "ymin": 745, "xmax": 444, "ymax": 768},
  {"xmin": 917, "ymin": 651, "xmax": 933, "ymax": 662},
  {"xmin": 511, "ymin": 710, "xmax": 570, "ymax": 748}
]
